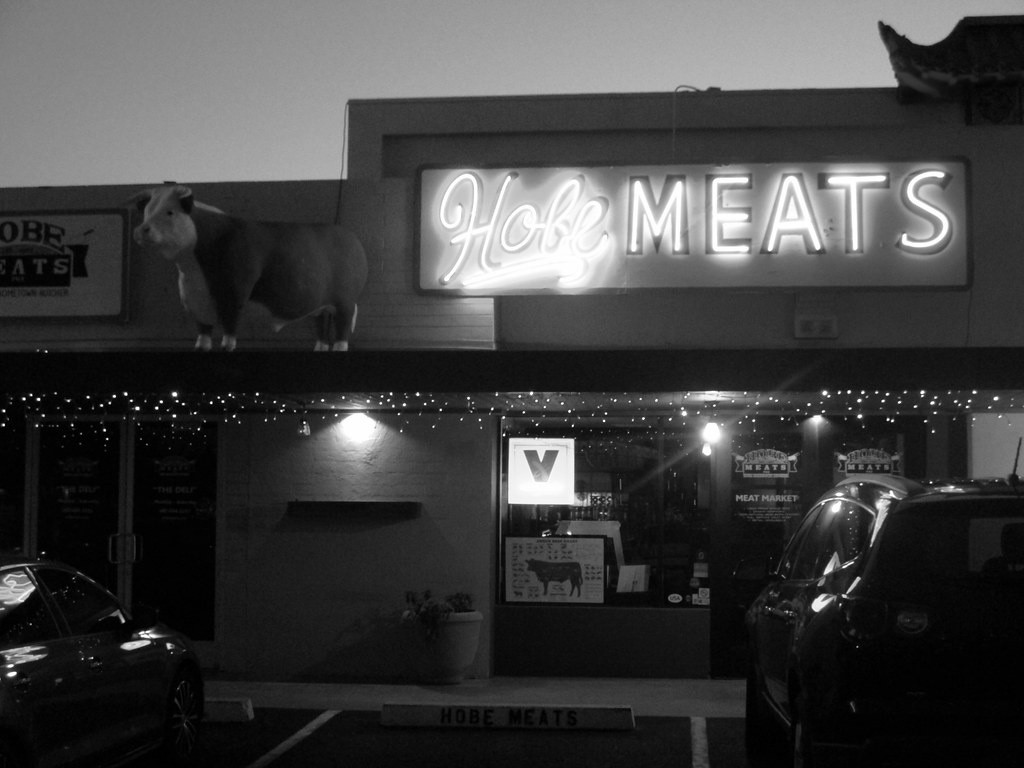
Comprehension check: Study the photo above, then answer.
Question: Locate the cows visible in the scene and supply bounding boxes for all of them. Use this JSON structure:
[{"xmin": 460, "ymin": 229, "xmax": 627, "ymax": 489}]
[{"xmin": 123, "ymin": 186, "xmax": 367, "ymax": 352}]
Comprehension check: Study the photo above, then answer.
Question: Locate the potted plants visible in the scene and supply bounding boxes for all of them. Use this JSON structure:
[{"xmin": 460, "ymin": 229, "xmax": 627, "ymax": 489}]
[{"xmin": 400, "ymin": 588, "xmax": 483, "ymax": 684}]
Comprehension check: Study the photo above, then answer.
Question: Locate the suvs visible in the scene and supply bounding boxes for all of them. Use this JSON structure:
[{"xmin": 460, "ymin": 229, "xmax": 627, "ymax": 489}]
[{"xmin": 745, "ymin": 474, "xmax": 1024, "ymax": 768}]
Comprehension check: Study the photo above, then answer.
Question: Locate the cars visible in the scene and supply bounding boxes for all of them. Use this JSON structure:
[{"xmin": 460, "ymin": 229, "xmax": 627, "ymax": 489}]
[{"xmin": 0, "ymin": 563, "xmax": 206, "ymax": 768}]
